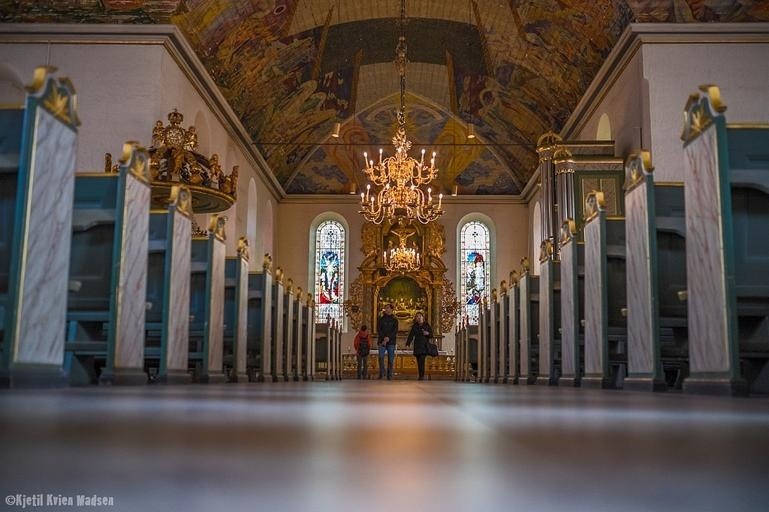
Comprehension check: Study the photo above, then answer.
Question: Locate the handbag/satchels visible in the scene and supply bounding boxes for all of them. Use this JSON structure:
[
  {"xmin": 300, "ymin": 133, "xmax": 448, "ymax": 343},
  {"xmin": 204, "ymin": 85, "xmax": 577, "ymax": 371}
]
[{"xmin": 426, "ymin": 342, "xmax": 438, "ymax": 357}]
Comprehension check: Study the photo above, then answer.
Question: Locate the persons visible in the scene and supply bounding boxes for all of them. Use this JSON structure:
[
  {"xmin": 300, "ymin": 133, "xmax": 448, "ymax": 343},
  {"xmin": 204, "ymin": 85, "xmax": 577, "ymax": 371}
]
[
  {"xmin": 354, "ymin": 325, "xmax": 372, "ymax": 380},
  {"xmin": 376, "ymin": 304, "xmax": 398, "ymax": 380},
  {"xmin": 405, "ymin": 312, "xmax": 438, "ymax": 380}
]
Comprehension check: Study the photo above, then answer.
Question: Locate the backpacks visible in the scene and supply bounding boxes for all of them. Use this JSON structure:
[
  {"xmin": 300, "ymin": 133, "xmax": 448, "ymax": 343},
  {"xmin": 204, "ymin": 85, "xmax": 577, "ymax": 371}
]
[{"xmin": 358, "ymin": 334, "xmax": 369, "ymax": 357}]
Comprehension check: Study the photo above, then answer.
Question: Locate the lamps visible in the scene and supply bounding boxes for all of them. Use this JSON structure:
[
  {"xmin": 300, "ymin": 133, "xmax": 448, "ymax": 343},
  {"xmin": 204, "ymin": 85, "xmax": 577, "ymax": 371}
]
[{"xmin": 355, "ymin": 1, "xmax": 444, "ymax": 274}]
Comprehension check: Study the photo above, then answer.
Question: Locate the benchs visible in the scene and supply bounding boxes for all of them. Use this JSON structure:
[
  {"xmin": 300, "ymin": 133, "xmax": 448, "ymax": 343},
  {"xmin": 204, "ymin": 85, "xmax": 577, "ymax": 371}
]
[
  {"xmin": 0, "ymin": 64, "xmax": 343, "ymax": 384},
  {"xmin": 453, "ymin": 83, "xmax": 768, "ymax": 396}
]
